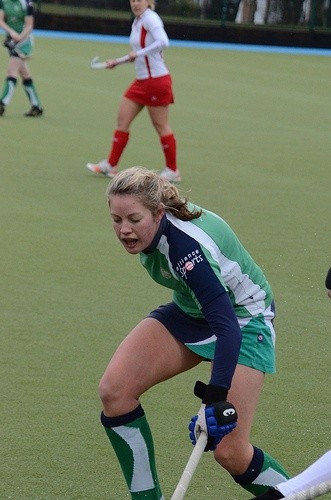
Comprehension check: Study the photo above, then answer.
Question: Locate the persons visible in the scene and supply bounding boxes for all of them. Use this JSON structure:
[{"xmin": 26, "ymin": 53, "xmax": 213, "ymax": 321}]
[
  {"xmin": 86, "ymin": 0, "xmax": 183, "ymax": 182},
  {"xmin": 0, "ymin": 0, "xmax": 44, "ymax": 117},
  {"xmin": 98, "ymin": 166, "xmax": 292, "ymax": 500}
]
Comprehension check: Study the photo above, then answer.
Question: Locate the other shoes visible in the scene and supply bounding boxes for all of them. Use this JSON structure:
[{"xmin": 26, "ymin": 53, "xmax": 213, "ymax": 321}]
[
  {"xmin": 25, "ymin": 106, "xmax": 43, "ymax": 117},
  {"xmin": 0, "ymin": 106, "xmax": 5, "ymax": 115}
]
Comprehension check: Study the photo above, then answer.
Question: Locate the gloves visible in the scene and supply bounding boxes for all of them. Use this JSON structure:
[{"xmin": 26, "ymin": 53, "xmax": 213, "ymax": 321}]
[{"xmin": 188, "ymin": 380, "xmax": 238, "ymax": 453}]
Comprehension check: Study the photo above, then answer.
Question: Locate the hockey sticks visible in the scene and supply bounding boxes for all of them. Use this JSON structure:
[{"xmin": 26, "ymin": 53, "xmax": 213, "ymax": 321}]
[
  {"xmin": 170, "ymin": 431, "xmax": 208, "ymax": 500},
  {"xmin": 276, "ymin": 479, "xmax": 331, "ymax": 500},
  {"xmin": 89, "ymin": 54, "xmax": 130, "ymax": 70}
]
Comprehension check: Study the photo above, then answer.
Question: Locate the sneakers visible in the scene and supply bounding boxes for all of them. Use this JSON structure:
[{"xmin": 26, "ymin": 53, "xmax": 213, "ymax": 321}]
[
  {"xmin": 159, "ymin": 166, "xmax": 181, "ymax": 183},
  {"xmin": 86, "ymin": 160, "xmax": 118, "ymax": 179}
]
